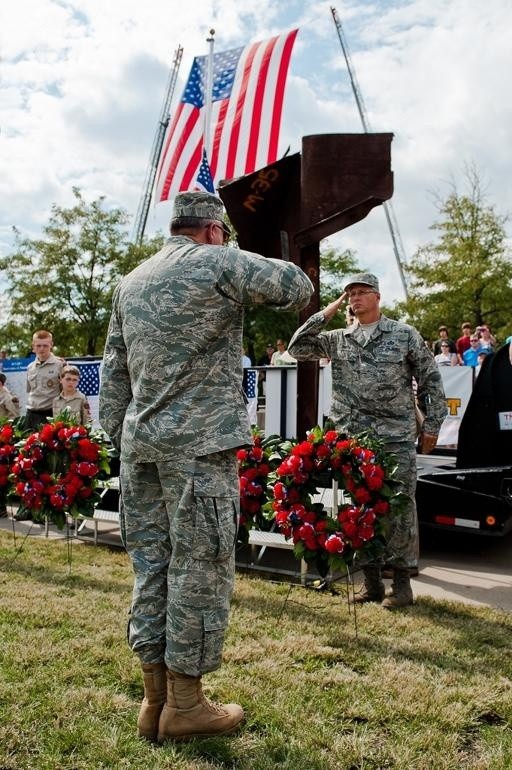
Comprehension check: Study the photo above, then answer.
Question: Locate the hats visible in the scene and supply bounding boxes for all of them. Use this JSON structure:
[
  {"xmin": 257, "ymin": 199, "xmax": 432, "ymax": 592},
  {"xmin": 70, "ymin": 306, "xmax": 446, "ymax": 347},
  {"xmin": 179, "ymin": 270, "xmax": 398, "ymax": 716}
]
[
  {"xmin": 462, "ymin": 322, "xmax": 471, "ymax": 329},
  {"xmin": 171, "ymin": 191, "xmax": 231, "ymax": 232}
]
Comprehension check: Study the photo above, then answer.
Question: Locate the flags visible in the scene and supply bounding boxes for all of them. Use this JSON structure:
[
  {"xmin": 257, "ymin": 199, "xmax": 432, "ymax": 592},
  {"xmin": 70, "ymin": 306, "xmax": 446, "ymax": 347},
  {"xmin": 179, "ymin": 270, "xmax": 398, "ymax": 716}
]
[{"xmin": 152, "ymin": 29, "xmax": 300, "ymax": 206}]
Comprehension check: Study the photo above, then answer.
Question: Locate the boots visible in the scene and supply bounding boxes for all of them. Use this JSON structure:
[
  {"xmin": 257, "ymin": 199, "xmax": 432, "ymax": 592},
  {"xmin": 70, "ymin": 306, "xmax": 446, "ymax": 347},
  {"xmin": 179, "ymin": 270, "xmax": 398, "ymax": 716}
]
[
  {"xmin": 381, "ymin": 568, "xmax": 414, "ymax": 607},
  {"xmin": 158, "ymin": 671, "xmax": 244, "ymax": 745},
  {"xmin": 350, "ymin": 567, "xmax": 385, "ymax": 601},
  {"xmin": 136, "ymin": 666, "xmax": 166, "ymax": 743}
]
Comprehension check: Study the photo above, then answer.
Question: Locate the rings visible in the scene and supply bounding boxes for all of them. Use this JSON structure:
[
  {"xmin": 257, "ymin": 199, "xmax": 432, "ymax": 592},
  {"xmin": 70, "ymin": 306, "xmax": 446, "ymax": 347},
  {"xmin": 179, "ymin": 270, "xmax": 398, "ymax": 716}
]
[{"xmin": 431, "ymin": 443, "xmax": 434, "ymax": 446}]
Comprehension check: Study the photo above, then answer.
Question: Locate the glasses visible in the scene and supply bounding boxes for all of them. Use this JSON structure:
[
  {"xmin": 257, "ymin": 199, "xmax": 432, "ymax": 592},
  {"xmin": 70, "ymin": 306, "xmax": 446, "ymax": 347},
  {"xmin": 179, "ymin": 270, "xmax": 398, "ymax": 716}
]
[
  {"xmin": 441, "ymin": 345, "xmax": 448, "ymax": 348},
  {"xmin": 344, "ymin": 273, "xmax": 379, "ymax": 291},
  {"xmin": 470, "ymin": 340, "xmax": 478, "ymax": 342},
  {"xmin": 348, "ymin": 290, "xmax": 375, "ymax": 297},
  {"xmin": 204, "ymin": 223, "xmax": 230, "ymax": 243}
]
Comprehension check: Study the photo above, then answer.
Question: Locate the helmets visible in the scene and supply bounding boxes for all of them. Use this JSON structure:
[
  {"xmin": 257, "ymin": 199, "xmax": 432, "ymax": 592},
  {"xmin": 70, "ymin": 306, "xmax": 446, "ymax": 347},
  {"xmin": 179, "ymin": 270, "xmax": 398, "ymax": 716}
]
[{"xmin": 438, "ymin": 326, "xmax": 449, "ymax": 337}]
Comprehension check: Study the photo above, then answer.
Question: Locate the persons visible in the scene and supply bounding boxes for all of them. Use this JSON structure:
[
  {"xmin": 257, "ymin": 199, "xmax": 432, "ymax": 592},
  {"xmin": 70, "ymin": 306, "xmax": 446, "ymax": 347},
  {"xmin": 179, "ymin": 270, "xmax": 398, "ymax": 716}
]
[
  {"xmin": 269, "ymin": 336, "xmax": 297, "ymax": 365},
  {"xmin": 285, "ymin": 272, "xmax": 449, "ymax": 611},
  {"xmin": 0, "ymin": 371, "xmax": 20, "ymax": 521},
  {"xmin": 52, "ymin": 363, "xmax": 93, "ymax": 531},
  {"xmin": 241, "ymin": 345, "xmax": 252, "ymax": 368},
  {"xmin": 15, "ymin": 330, "xmax": 71, "ymax": 524},
  {"xmin": 96, "ymin": 186, "xmax": 314, "ymax": 743},
  {"xmin": 257, "ymin": 341, "xmax": 274, "ymax": 365},
  {"xmin": 425, "ymin": 321, "xmax": 496, "ymax": 370}
]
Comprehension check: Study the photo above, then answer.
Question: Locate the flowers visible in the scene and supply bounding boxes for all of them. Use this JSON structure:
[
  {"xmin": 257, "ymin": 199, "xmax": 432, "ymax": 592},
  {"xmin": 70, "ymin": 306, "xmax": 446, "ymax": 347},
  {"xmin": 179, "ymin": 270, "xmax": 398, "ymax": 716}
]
[
  {"xmin": 261, "ymin": 423, "xmax": 414, "ymax": 577},
  {"xmin": 6, "ymin": 406, "xmax": 111, "ymax": 530},
  {"xmin": 237, "ymin": 423, "xmax": 300, "ymax": 532},
  {"xmin": 0, "ymin": 415, "xmax": 36, "ymax": 486}
]
